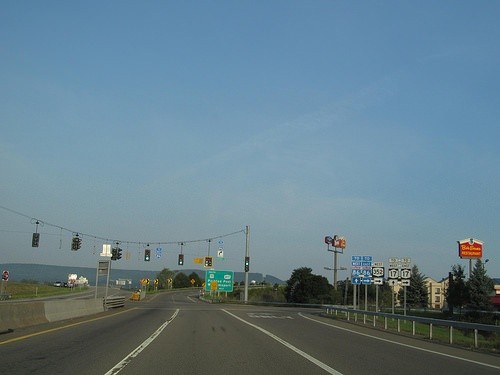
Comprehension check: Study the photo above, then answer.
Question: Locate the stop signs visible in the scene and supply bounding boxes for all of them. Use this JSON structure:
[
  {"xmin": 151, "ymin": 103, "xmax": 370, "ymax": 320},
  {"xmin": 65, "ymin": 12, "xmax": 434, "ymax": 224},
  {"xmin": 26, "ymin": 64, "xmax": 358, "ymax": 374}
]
[{"xmin": 2, "ymin": 271, "xmax": 9, "ymax": 280}]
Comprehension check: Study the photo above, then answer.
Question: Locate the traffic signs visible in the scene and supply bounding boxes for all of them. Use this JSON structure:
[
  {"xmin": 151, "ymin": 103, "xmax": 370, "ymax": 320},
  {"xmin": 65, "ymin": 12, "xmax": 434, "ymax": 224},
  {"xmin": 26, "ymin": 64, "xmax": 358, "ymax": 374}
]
[{"xmin": 205, "ymin": 270, "xmax": 235, "ymax": 292}]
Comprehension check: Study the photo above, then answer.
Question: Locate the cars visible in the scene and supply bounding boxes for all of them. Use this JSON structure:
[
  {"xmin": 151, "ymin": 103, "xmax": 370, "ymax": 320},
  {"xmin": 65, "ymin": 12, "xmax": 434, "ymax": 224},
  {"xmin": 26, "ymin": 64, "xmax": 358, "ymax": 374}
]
[
  {"xmin": 60, "ymin": 282, "xmax": 69, "ymax": 287},
  {"xmin": 54, "ymin": 281, "xmax": 63, "ymax": 287}
]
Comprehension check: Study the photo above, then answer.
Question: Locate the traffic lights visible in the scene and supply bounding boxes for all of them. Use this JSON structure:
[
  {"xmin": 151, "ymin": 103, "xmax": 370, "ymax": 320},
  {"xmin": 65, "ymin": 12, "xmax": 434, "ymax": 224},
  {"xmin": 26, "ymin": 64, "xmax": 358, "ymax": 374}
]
[
  {"xmin": 71, "ymin": 237, "xmax": 82, "ymax": 251},
  {"xmin": 32, "ymin": 232, "xmax": 39, "ymax": 247},
  {"xmin": 204, "ymin": 257, "xmax": 213, "ymax": 266},
  {"xmin": 178, "ymin": 254, "xmax": 184, "ymax": 265},
  {"xmin": 245, "ymin": 257, "xmax": 250, "ymax": 272},
  {"xmin": 116, "ymin": 247, "xmax": 122, "ymax": 259},
  {"xmin": 144, "ymin": 250, "xmax": 151, "ymax": 260},
  {"xmin": 111, "ymin": 249, "xmax": 117, "ymax": 260}
]
